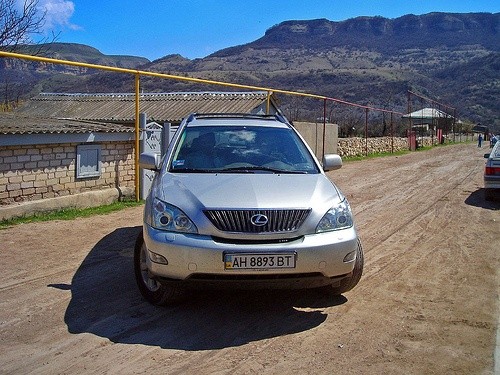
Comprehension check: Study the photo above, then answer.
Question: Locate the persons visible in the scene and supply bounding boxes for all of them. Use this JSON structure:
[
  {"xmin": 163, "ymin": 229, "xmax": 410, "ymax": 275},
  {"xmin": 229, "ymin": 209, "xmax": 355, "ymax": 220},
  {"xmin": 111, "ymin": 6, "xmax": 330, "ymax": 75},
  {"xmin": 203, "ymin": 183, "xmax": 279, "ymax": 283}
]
[{"xmin": 478, "ymin": 133, "xmax": 482, "ymax": 147}]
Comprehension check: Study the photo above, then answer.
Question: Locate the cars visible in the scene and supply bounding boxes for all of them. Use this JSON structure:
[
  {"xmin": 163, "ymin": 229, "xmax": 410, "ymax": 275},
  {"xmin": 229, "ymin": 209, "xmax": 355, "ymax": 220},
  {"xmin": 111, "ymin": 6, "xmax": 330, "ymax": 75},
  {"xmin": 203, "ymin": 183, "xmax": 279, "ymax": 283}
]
[
  {"xmin": 134, "ymin": 114, "xmax": 364, "ymax": 304},
  {"xmin": 483, "ymin": 140, "xmax": 500, "ymax": 201}
]
